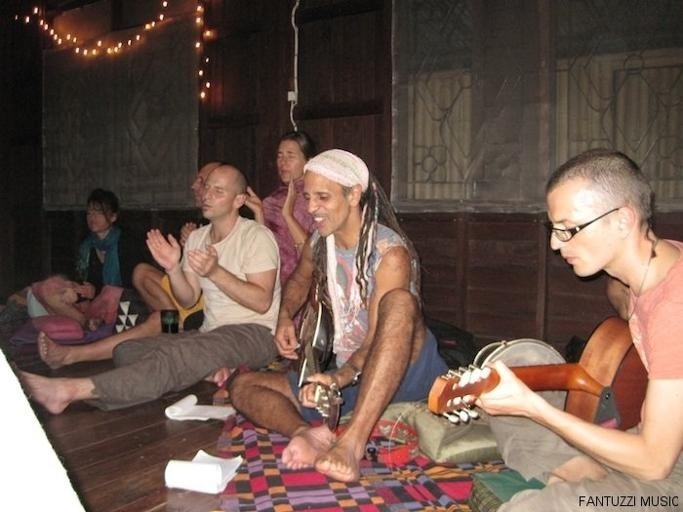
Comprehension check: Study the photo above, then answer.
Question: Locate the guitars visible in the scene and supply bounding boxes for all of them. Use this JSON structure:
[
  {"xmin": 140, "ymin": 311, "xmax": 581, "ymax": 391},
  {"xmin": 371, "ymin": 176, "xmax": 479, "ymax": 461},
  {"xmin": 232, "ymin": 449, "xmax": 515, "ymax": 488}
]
[
  {"xmin": 292, "ymin": 283, "xmax": 344, "ymax": 433},
  {"xmin": 427, "ymin": 316, "xmax": 648, "ymax": 431}
]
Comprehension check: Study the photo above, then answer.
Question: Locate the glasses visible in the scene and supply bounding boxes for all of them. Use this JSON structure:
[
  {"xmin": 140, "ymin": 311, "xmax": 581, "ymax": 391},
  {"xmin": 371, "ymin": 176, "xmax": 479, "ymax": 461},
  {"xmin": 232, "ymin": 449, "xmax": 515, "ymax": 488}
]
[{"xmin": 543, "ymin": 206, "xmax": 621, "ymax": 242}]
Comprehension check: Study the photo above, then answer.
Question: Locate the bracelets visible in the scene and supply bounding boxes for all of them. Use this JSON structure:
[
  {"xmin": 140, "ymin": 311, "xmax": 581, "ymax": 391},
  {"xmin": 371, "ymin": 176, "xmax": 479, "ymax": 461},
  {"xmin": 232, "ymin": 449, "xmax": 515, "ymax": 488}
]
[
  {"xmin": 293, "ymin": 239, "xmax": 308, "ymax": 249},
  {"xmin": 344, "ymin": 360, "xmax": 362, "ymax": 385}
]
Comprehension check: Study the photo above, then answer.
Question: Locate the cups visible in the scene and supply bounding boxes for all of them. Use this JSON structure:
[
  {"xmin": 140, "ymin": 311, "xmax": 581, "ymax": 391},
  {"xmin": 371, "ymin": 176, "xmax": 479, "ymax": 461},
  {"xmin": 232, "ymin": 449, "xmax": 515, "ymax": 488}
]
[{"xmin": 160, "ymin": 309, "xmax": 180, "ymax": 334}]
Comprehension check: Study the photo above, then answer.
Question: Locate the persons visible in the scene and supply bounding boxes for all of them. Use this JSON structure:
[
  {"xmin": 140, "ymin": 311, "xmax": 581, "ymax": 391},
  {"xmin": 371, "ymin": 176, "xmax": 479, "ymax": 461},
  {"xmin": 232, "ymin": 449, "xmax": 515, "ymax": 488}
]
[
  {"xmin": 36, "ymin": 159, "xmax": 222, "ymax": 371},
  {"xmin": 8, "ymin": 166, "xmax": 283, "ymax": 416},
  {"xmin": 469, "ymin": 145, "xmax": 683, "ymax": 511},
  {"xmin": 225, "ymin": 147, "xmax": 449, "ymax": 486},
  {"xmin": 36, "ymin": 186, "xmax": 148, "ymax": 333},
  {"xmin": 240, "ymin": 130, "xmax": 318, "ymax": 289}
]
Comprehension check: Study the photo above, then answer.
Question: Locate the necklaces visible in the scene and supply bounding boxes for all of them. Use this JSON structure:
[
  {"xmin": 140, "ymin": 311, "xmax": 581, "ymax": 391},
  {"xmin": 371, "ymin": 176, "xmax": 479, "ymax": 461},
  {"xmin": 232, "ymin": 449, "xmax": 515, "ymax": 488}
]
[{"xmin": 622, "ymin": 235, "xmax": 660, "ymax": 328}]
